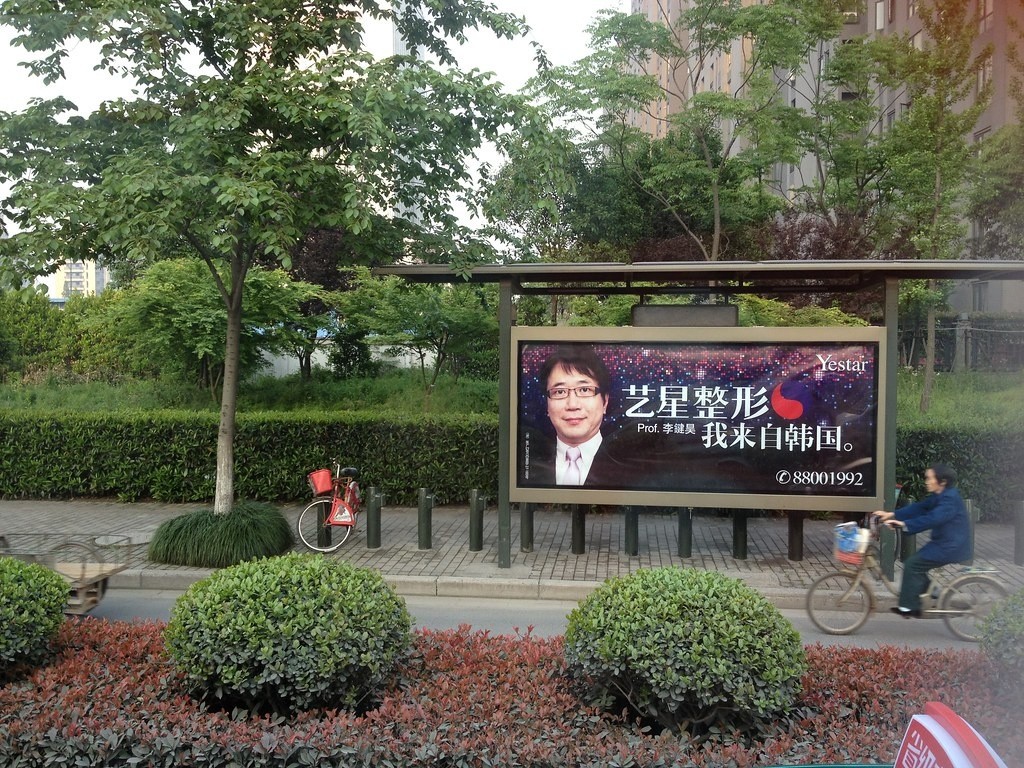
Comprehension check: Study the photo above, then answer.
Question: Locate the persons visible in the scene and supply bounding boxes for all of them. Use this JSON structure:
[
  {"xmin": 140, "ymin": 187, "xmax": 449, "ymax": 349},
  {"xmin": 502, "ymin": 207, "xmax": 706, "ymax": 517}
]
[
  {"xmin": 524, "ymin": 345, "xmax": 636, "ymax": 486},
  {"xmin": 872, "ymin": 463, "xmax": 973, "ymax": 618}
]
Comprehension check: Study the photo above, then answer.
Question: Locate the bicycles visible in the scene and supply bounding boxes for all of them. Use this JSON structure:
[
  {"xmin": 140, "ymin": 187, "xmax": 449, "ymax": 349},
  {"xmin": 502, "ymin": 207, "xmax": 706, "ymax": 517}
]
[
  {"xmin": 297, "ymin": 454, "xmax": 363, "ymax": 554},
  {"xmin": 807, "ymin": 514, "xmax": 1010, "ymax": 644}
]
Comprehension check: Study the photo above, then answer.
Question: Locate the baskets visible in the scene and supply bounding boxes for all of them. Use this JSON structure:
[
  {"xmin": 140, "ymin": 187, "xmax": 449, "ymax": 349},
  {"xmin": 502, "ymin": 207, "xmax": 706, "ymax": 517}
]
[
  {"xmin": 308, "ymin": 469, "xmax": 332, "ymax": 497},
  {"xmin": 833, "ymin": 529, "xmax": 870, "ymax": 564}
]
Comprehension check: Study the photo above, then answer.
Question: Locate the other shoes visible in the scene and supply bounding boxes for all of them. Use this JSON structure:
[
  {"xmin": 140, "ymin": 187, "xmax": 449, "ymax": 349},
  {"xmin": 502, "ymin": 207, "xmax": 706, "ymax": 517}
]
[{"xmin": 891, "ymin": 606, "xmax": 923, "ymax": 619}]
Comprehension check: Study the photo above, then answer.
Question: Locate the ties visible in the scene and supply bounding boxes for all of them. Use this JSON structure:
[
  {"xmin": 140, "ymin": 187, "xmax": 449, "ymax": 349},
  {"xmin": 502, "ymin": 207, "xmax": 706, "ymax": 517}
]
[{"xmin": 563, "ymin": 447, "xmax": 581, "ymax": 485}]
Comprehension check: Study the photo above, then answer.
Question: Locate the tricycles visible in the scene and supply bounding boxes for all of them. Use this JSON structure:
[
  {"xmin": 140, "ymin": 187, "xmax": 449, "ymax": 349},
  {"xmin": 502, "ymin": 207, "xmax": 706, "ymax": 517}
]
[{"xmin": 0, "ymin": 529, "xmax": 132, "ymax": 623}]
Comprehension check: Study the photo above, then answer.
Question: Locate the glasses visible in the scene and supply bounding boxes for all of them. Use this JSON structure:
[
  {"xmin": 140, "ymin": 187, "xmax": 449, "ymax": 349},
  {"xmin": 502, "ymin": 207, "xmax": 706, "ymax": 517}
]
[{"xmin": 546, "ymin": 386, "xmax": 601, "ymax": 399}]
